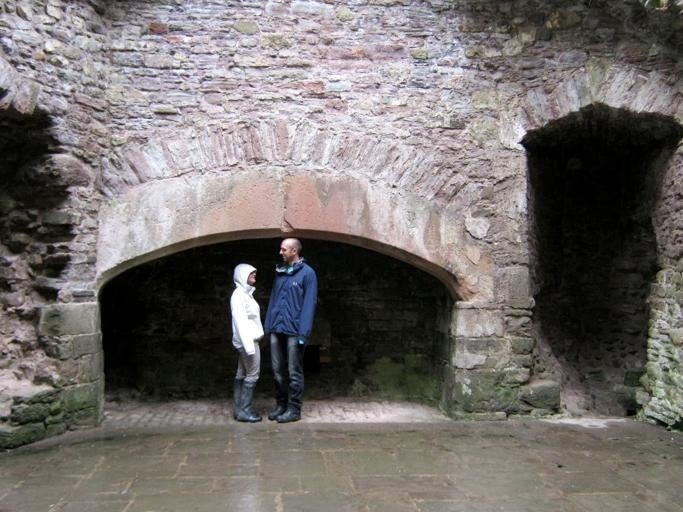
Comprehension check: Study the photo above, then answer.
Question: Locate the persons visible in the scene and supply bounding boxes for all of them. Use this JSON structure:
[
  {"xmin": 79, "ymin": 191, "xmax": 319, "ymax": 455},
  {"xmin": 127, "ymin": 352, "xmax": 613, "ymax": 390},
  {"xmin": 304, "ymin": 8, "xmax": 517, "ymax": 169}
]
[
  {"xmin": 262, "ymin": 237, "xmax": 319, "ymax": 422},
  {"xmin": 228, "ymin": 263, "xmax": 265, "ymax": 424}
]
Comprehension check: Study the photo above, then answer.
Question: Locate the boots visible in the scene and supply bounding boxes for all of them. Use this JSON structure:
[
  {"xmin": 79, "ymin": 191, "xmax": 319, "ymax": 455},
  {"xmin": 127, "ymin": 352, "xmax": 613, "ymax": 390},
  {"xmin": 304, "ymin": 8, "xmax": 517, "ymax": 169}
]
[
  {"xmin": 237, "ymin": 381, "xmax": 263, "ymax": 422},
  {"xmin": 276, "ymin": 405, "xmax": 301, "ymax": 423},
  {"xmin": 234, "ymin": 378, "xmax": 244, "ymax": 418},
  {"xmin": 268, "ymin": 402, "xmax": 286, "ymax": 420}
]
